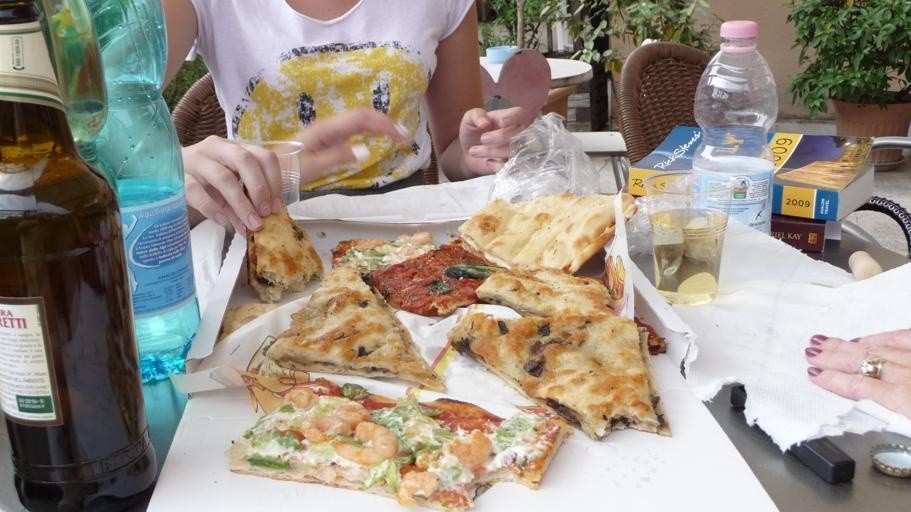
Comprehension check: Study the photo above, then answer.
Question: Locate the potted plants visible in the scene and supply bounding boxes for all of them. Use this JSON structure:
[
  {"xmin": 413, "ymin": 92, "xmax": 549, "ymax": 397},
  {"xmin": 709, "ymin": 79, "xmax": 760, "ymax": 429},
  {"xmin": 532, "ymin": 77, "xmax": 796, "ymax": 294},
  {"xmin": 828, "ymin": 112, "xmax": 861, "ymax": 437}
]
[{"xmin": 784, "ymin": 4, "xmax": 910, "ymax": 167}]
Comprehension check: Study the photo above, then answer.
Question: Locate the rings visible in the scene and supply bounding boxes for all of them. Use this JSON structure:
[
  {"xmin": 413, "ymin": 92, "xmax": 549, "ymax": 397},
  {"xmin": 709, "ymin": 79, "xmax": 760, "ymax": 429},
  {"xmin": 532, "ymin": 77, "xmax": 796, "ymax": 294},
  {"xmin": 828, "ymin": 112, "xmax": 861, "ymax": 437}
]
[{"xmin": 864, "ymin": 355, "xmax": 889, "ymax": 380}]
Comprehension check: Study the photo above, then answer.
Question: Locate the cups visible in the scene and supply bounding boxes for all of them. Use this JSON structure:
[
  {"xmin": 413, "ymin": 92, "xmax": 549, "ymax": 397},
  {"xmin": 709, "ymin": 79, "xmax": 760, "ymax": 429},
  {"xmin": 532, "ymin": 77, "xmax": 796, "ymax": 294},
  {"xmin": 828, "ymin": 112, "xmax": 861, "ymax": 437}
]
[
  {"xmin": 240, "ymin": 141, "xmax": 305, "ymax": 206},
  {"xmin": 643, "ymin": 166, "xmax": 735, "ymax": 308}
]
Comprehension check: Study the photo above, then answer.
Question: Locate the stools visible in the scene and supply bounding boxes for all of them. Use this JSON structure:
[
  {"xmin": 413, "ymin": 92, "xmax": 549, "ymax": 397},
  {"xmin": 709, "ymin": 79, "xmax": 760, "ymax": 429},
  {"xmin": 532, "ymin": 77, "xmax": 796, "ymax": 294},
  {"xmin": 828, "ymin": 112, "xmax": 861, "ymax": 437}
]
[{"xmin": 567, "ymin": 131, "xmax": 627, "ymax": 194}]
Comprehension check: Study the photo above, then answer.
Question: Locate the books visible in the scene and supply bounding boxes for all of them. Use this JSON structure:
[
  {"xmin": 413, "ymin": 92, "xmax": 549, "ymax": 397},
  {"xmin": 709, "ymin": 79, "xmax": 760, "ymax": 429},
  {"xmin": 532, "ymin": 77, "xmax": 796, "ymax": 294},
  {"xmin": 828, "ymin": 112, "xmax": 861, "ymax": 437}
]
[{"xmin": 620, "ymin": 123, "xmax": 876, "ymax": 258}]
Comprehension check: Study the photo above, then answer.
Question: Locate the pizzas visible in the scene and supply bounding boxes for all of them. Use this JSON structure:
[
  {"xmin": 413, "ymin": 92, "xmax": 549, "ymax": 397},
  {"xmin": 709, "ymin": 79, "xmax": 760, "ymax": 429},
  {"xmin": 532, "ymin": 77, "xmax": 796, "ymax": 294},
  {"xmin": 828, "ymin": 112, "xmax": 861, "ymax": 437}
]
[
  {"xmin": 459, "ymin": 192, "xmax": 638, "ymax": 277},
  {"xmin": 218, "ymin": 304, "xmax": 276, "ymax": 343},
  {"xmin": 264, "ymin": 279, "xmax": 445, "ymax": 391},
  {"xmin": 452, "ymin": 313, "xmax": 670, "ymax": 442},
  {"xmin": 327, "ymin": 232, "xmax": 495, "ymax": 321},
  {"xmin": 229, "ymin": 378, "xmax": 566, "ymax": 511},
  {"xmin": 474, "ymin": 267, "xmax": 611, "ymax": 318},
  {"xmin": 242, "ymin": 181, "xmax": 323, "ymax": 303}
]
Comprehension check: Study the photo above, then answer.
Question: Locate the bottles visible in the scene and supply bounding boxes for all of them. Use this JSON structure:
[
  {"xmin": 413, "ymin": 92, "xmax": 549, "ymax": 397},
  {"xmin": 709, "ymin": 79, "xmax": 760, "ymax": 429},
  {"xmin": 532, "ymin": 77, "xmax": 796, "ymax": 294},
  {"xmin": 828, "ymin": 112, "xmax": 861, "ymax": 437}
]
[
  {"xmin": 2, "ymin": 2, "xmax": 159, "ymax": 507},
  {"xmin": 84, "ymin": 2, "xmax": 203, "ymax": 388},
  {"xmin": 691, "ymin": 19, "xmax": 779, "ymax": 250},
  {"xmin": 38, "ymin": 2, "xmax": 115, "ymax": 207}
]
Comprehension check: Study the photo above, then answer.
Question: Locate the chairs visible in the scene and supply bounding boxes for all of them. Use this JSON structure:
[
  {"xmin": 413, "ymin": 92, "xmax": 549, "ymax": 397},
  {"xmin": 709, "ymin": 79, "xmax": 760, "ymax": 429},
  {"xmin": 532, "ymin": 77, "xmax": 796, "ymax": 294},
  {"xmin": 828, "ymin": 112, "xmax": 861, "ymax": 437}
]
[
  {"xmin": 621, "ymin": 40, "xmax": 712, "ymax": 170},
  {"xmin": 169, "ymin": 72, "xmax": 229, "ymax": 147},
  {"xmin": 838, "ymin": 193, "xmax": 911, "ymax": 260}
]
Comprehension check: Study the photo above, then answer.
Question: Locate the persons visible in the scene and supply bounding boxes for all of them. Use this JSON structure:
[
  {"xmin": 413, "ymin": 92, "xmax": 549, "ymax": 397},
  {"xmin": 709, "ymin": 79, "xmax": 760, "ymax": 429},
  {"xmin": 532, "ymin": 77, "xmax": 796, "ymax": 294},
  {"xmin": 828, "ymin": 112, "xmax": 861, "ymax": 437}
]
[
  {"xmin": 805, "ymin": 327, "xmax": 911, "ymax": 423},
  {"xmin": 161, "ymin": 0, "xmax": 523, "ymax": 232},
  {"xmin": 188, "ymin": 108, "xmax": 411, "ymax": 231}
]
